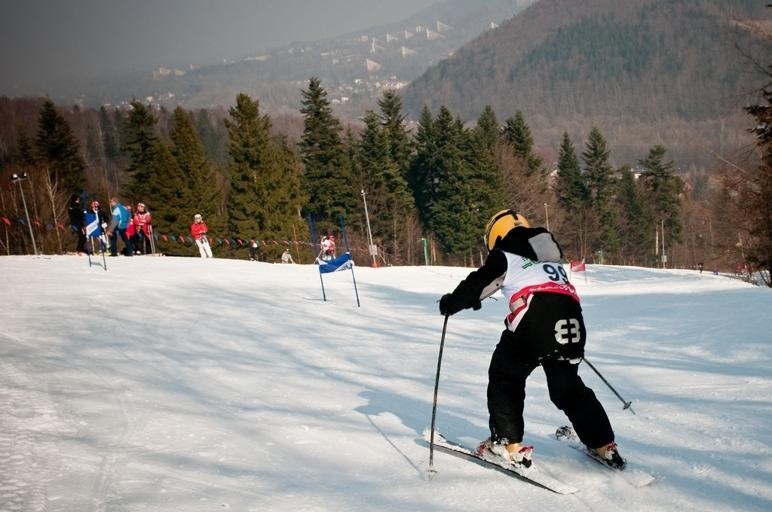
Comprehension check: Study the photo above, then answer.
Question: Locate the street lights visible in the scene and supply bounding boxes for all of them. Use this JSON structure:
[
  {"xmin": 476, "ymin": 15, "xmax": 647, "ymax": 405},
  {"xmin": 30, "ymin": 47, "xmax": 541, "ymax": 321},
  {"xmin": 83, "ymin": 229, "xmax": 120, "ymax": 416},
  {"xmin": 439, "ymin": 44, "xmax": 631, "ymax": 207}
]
[
  {"xmin": 10, "ymin": 172, "xmax": 39, "ymax": 255},
  {"xmin": 544, "ymin": 202, "xmax": 550, "ymax": 232},
  {"xmin": 360, "ymin": 189, "xmax": 379, "ymax": 268}
]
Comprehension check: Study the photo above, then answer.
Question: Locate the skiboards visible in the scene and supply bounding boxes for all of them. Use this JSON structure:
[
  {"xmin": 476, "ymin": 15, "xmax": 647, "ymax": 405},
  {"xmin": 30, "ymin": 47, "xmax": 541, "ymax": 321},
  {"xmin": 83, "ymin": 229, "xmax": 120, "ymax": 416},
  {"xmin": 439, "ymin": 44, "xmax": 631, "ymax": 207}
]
[{"xmin": 422, "ymin": 424, "xmax": 655, "ymax": 493}]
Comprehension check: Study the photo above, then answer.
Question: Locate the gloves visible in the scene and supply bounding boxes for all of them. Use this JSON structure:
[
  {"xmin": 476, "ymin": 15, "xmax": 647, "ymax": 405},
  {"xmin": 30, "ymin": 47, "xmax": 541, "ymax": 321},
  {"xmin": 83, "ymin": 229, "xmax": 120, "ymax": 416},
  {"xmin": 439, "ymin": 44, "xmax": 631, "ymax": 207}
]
[{"xmin": 439, "ymin": 293, "xmax": 455, "ymax": 314}]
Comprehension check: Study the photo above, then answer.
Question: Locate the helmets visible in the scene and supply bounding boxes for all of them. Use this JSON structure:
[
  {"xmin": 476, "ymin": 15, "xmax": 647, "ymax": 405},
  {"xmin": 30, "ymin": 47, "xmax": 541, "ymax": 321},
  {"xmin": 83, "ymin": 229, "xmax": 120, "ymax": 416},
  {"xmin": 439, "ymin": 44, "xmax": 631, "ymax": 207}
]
[{"xmin": 483, "ymin": 209, "xmax": 531, "ymax": 252}]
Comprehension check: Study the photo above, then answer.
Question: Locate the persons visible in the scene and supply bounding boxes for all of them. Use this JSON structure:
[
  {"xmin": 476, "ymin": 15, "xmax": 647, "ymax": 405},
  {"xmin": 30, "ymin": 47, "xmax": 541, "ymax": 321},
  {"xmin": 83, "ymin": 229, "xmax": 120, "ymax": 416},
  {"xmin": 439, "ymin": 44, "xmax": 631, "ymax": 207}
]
[
  {"xmin": 328, "ymin": 236, "xmax": 335, "ymax": 260},
  {"xmin": 439, "ymin": 205, "xmax": 626, "ymax": 473},
  {"xmin": 248, "ymin": 236, "xmax": 261, "ymax": 260},
  {"xmin": 67, "ymin": 195, "xmax": 153, "ymax": 256},
  {"xmin": 281, "ymin": 247, "xmax": 295, "ymax": 263},
  {"xmin": 320, "ymin": 235, "xmax": 329, "ymax": 260},
  {"xmin": 698, "ymin": 263, "xmax": 703, "ymax": 273},
  {"xmin": 190, "ymin": 214, "xmax": 213, "ymax": 257}
]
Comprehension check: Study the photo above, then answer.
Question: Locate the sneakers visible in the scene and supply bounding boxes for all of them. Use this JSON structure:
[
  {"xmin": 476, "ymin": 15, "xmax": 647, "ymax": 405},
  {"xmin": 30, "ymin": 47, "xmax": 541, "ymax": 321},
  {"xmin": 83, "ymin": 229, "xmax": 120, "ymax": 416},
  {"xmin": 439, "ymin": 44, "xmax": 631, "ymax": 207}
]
[
  {"xmin": 476, "ymin": 440, "xmax": 524, "ymax": 461},
  {"xmin": 591, "ymin": 441, "xmax": 616, "ymax": 462}
]
